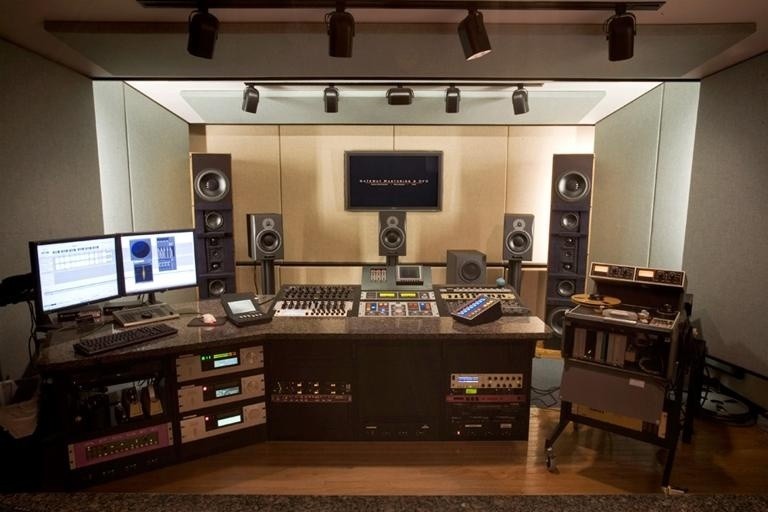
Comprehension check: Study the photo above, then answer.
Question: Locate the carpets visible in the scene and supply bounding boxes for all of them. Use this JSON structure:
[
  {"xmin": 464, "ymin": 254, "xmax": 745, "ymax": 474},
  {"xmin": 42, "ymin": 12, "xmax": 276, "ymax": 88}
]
[{"xmin": 525, "ymin": 353, "xmax": 565, "ymax": 411}]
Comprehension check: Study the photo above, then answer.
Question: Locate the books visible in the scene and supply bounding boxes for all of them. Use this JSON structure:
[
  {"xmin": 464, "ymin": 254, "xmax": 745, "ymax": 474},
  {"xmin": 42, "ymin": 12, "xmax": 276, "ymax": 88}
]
[{"xmin": 566, "ymin": 327, "xmax": 627, "ymax": 365}]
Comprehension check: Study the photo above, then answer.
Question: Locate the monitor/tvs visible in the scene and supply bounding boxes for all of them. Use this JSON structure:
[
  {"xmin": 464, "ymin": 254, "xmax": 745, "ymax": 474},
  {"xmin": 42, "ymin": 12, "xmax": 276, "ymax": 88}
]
[
  {"xmin": 202, "ymin": 305, "xmax": 207, "ymax": 306},
  {"xmin": 343, "ymin": 149, "xmax": 444, "ymax": 212},
  {"xmin": 28, "ymin": 233, "xmax": 123, "ymax": 318},
  {"xmin": 118, "ymin": 230, "xmax": 200, "ymax": 307}
]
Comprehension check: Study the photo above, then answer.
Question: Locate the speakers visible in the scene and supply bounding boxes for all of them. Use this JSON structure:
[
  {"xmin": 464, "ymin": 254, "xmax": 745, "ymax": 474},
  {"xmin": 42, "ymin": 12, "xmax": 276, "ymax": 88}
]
[
  {"xmin": 377, "ymin": 212, "xmax": 407, "ymax": 256},
  {"xmin": 246, "ymin": 213, "xmax": 284, "ymax": 260},
  {"xmin": 551, "ymin": 152, "xmax": 594, "ymax": 212},
  {"xmin": 544, "ymin": 297, "xmax": 584, "ymax": 350},
  {"xmin": 195, "ymin": 209, "xmax": 236, "ymax": 300},
  {"xmin": 447, "ymin": 249, "xmax": 488, "ymax": 284},
  {"xmin": 503, "ymin": 212, "xmax": 535, "ymax": 262},
  {"xmin": 189, "ymin": 153, "xmax": 232, "ymax": 208},
  {"xmin": 547, "ymin": 211, "xmax": 590, "ymax": 296}
]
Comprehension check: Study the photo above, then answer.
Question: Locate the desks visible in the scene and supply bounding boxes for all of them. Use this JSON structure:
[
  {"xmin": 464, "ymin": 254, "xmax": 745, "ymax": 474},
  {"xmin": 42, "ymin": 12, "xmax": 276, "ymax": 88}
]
[{"xmin": 35, "ymin": 284, "xmax": 555, "ymax": 492}]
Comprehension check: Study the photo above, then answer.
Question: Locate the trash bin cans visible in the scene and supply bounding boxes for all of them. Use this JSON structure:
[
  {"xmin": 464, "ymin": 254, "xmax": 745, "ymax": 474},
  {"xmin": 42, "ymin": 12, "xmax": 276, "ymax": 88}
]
[{"xmin": 530, "ymin": 358, "xmax": 564, "ymax": 404}]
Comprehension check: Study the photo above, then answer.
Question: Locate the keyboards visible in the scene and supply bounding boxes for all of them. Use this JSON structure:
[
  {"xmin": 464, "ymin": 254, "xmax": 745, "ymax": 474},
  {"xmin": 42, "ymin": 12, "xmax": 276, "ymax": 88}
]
[
  {"xmin": 113, "ymin": 302, "xmax": 181, "ymax": 328},
  {"xmin": 73, "ymin": 320, "xmax": 177, "ymax": 355}
]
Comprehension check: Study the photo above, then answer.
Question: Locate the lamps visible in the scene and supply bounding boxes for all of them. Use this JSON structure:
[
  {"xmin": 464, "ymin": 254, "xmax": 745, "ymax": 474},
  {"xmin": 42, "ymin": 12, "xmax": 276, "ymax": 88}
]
[
  {"xmin": 242, "ymin": 81, "xmax": 532, "ymax": 117},
  {"xmin": 184, "ymin": 6, "xmax": 640, "ymax": 65}
]
